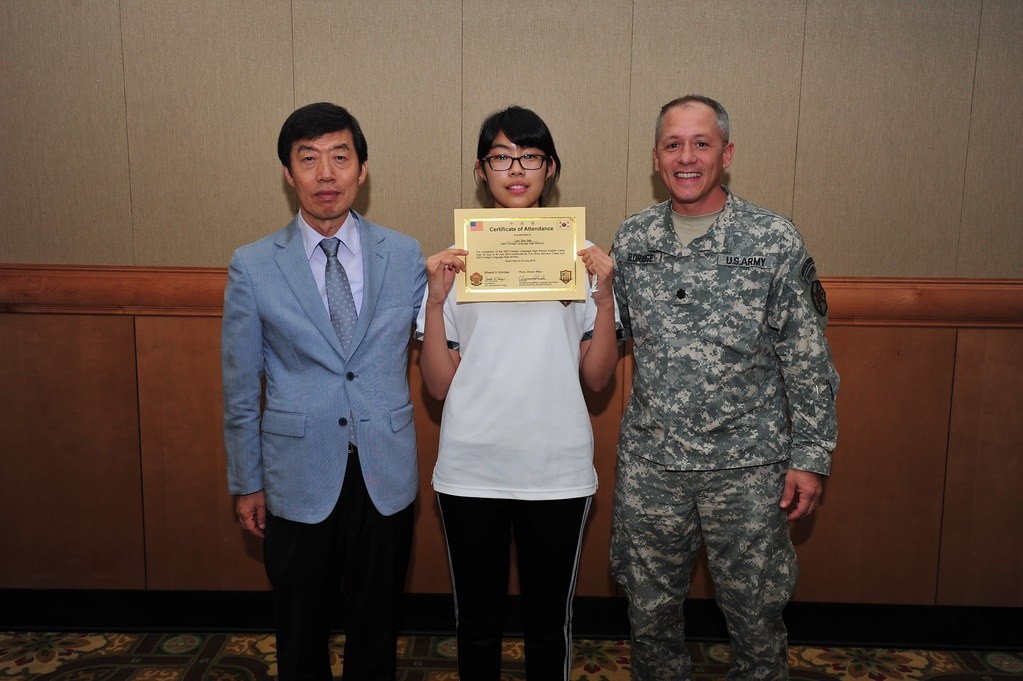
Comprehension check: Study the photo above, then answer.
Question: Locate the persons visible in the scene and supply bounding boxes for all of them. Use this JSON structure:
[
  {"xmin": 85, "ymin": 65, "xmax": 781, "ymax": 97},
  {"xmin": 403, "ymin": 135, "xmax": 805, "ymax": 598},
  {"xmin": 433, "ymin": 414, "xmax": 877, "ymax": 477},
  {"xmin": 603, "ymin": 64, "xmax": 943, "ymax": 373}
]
[
  {"xmin": 221, "ymin": 103, "xmax": 428, "ymax": 681},
  {"xmin": 414, "ymin": 105, "xmax": 625, "ymax": 681},
  {"xmin": 608, "ymin": 95, "xmax": 840, "ymax": 680}
]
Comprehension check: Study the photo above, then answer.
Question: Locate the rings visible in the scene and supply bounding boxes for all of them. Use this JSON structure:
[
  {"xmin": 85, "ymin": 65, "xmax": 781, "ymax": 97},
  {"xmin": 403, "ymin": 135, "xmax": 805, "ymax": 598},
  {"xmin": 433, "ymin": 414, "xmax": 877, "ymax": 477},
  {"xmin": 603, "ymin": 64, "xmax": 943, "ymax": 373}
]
[{"xmin": 444, "ymin": 265, "xmax": 448, "ymax": 268}]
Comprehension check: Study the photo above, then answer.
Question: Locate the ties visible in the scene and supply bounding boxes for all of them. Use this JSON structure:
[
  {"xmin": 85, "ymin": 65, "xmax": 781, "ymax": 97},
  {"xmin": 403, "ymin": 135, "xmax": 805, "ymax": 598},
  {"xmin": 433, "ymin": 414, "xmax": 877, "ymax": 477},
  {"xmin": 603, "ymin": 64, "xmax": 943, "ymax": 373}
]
[{"xmin": 318, "ymin": 237, "xmax": 358, "ymax": 358}]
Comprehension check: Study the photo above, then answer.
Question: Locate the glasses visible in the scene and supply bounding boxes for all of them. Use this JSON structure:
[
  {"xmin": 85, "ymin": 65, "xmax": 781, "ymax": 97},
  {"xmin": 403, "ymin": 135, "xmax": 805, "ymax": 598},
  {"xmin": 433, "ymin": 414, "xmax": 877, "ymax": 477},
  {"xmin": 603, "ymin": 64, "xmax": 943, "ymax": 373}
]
[{"xmin": 482, "ymin": 154, "xmax": 548, "ymax": 171}]
